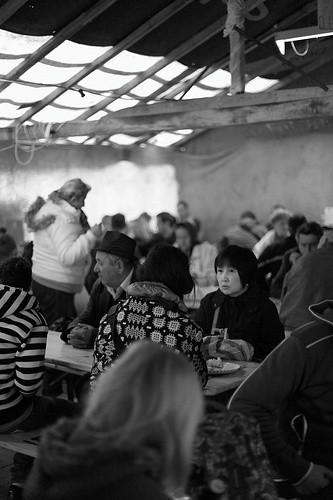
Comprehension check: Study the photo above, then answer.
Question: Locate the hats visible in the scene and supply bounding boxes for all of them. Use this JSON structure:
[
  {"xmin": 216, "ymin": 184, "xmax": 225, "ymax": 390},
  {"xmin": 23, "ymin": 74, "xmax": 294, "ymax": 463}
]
[
  {"xmin": 137, "ymin": 244, "xmax": 195, "ymax": 294},
  {"xmin": 92, "ymin": 230, "xmax": 137, "ymax": 261}
]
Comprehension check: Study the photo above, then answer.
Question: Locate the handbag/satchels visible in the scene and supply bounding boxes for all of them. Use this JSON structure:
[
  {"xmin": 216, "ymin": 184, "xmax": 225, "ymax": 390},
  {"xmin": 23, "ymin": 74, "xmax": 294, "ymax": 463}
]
[{"xmin": 186, "ymin": 401, "xmax": 272, "ymax": 500}]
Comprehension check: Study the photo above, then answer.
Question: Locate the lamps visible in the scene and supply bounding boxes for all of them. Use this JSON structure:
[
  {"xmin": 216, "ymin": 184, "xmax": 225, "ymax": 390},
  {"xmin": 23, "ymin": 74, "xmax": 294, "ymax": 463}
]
[{"xmin": 274, "ymin": 27, "xmax": 333, "ymax": 55}]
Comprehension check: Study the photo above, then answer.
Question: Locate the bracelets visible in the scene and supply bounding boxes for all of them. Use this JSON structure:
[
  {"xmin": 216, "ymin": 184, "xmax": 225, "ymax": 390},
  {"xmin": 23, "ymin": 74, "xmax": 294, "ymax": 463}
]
[{"xmin": 292, "ymin": 462, "xmax": 314, "ymax": 486}]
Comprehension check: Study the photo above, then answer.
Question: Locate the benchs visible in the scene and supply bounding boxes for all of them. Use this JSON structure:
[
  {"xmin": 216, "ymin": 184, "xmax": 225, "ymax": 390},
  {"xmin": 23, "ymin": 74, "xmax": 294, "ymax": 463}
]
[{"xmin": 0, "ymin": 424, "xmax": 55, "ymax": 458}]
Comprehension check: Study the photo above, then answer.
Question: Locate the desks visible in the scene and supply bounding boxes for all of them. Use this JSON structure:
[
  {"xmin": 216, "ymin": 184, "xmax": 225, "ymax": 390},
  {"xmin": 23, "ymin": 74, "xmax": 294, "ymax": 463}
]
[{"xmin": 45, "ymin": 330, "xmax": 262, "ymax": 398}]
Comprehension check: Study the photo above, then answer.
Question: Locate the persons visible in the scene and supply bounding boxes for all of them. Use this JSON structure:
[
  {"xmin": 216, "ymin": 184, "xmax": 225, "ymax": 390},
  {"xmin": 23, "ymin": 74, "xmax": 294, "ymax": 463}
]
[
  {"xmin": 24, "ymin": 179, "xmax": 102, "ymax": 401},
  {"xmin": 0, "ymin": 201, "xmax": 333, "ymax": 500}
]
